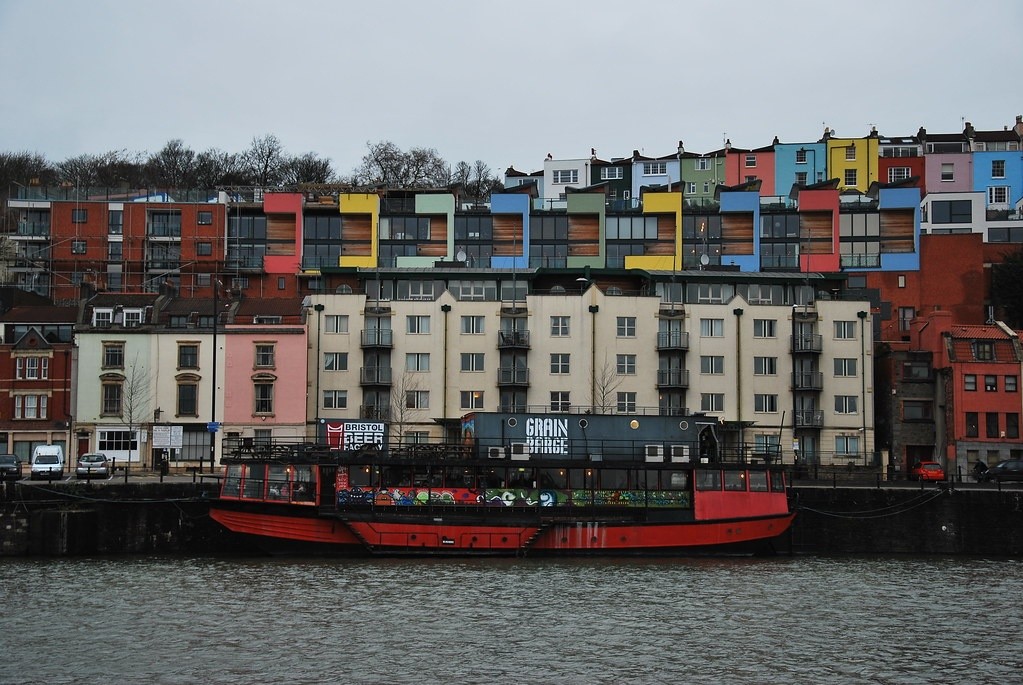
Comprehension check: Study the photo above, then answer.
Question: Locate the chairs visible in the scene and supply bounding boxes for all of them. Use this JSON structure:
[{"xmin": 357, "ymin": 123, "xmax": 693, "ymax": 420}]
[
  {"xmin": 500, "ymin": 331, "xmax": 527, "ymax": 345},
  {"xmin": 95, "ymin": 458, "xmax": 102, "ymax": 462}
]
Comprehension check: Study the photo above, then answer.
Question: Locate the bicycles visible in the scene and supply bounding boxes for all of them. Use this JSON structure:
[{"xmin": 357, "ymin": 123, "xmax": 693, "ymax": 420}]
[{"xmin": 966, "ymin": 469, "xmax": 983, "ymax": 484}]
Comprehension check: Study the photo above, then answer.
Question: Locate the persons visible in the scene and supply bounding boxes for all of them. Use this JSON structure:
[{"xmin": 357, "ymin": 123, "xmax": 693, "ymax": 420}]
[
  {"xmin": 269, "ymin": 483, "xmax": 278, "ymax": 495},
  {"xmin": 974, "ymin": 459, "xmax": 983, "ymax": 484},
  {"xmin": 281, "ymin": 484, "xmax": 289, "ymax": 496},
  {"xmin": 296, "ymin": 483, "xmax": 305, "ymax": 492}
]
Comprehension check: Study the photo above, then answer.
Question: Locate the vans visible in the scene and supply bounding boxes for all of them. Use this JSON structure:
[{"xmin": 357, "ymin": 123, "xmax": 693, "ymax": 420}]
[{"xmin": 28, "ymin": 445, "xmax": 66, "ymax": 481}]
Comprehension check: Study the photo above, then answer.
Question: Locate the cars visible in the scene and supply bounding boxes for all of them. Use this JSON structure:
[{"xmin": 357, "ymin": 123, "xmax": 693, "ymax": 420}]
[
  {"xmin": 0, "ymin": 454, "xmax": 22, "ymax": 481},
  {"xmin": 76, "ymin": 453, "xmax": 112, "ymax": 479},
  {"xmin": 983, "ymin": 459, "xmax": 1023, "ymax": 482},
  {"xmin": 911, "ymin": 461, "xmax": 944, "ymax": 483}
]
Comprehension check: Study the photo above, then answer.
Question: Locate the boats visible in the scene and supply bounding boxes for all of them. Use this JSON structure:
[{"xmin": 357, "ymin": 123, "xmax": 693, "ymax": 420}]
[{"xmin": 203, "ymin": 436, "xmax": 800, "ymax": 559}]
